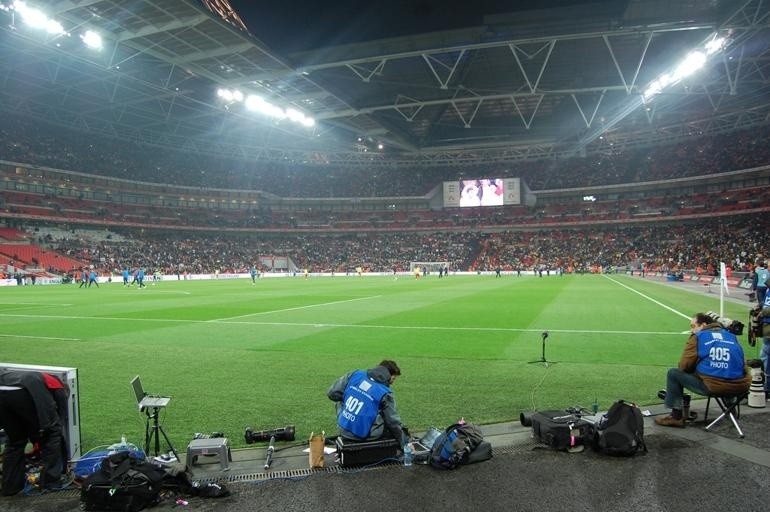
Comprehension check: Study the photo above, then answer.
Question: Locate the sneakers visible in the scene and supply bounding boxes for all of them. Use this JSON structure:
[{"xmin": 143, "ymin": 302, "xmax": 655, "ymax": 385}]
[
  {"xmin": 50, "ymin": 470, "xmax": 75, "ymax": 491},
  {"xmin": 725, "ymin": 407, "xmax": 738, "ymax": 416},
  {"xmin": 654, "ymin": 414, "xmax": 682, "ymax": 426}
]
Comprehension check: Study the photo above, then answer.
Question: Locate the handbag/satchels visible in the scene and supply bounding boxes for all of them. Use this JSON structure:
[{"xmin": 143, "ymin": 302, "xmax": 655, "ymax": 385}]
[
  {"xmin": 425, "ymin": 418, "xmax": 485, "ymax": 470},
  {"xmin": 79, "ymin": 463, "xmax": 162, "ymax": 512},
  {"xmin": 748, "ymin": 310, "xmax": 762, "ymax": 346}
]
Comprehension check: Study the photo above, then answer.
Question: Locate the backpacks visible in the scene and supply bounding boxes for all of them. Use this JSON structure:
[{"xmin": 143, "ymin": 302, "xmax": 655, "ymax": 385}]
[{"xmin": 590, "ymin": 399, "xmax": 648, "ymax": 456}]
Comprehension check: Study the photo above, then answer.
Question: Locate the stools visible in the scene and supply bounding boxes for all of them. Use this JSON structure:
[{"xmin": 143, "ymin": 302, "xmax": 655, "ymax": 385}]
[
  {"xmin": 186, "ymin": 437, "xmax": 233, "ymax": 473},
  {"xmin": 705, "ymin": 391, "xmax": 748, "ymax": 440}
]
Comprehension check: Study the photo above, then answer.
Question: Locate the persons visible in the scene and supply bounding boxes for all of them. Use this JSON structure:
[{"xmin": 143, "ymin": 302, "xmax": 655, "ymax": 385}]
[
  {"xmin": 326, "ymin": 359, "xmax": 418, "ymax": 451},
  {"xmin": 529, "ymin": 124, "xmax": 769, "ymax": 280},
  {"xmin": 130, "ymin": 269, "xmax": 141, "ymax": 286},
  {"xmin": 751, "ymin": 261, "xmax": 770, "ymax": 304},
  {"xmin": 453, "ymin": 212, "xmax": 538, "ymax": 278},
  {"xmin": 753, "ymin": 285, "xmax": 770, "ymax": 402},
  {"xmin": 88, "ymin": 270, "xmax": 99, "ymax": 288},
  {"xmin": 460, "ymin": 178, "xmax": 502, "ymax": 206},
  {"xmin": 122, "ymin": 267, "xmax": 130, "ymax": 288},
  {"xmin": 77, "ymin": 270, "xmax": 88, "ymax": 288},
  {"xmin": 249, "ymin": 265, "xmax": 257, "ymax": 285},
  {"xmin": 0, "ymin": 110, "xmax": 458, "ymax": 279},
  {"xmin": 31, "ymin": 272, "xmax": 36, "ymax": 286},
  {"xmin": 152, "ymin": 270, "xmax": 159, "ymax": 286},
  {"xmin": 138, "ymin": 267, "xmax": 147, "ymax": 289},
  {"xmin": 748, "ymin": 254, "xmax": 765, "ymax": 292},
  {"xmin": 653, "ymin": 313, "xmax": 753, "ymax": 429},
  {"xmin": 455, "ymin": 160, "xmax": 532, "ymax": 179}
]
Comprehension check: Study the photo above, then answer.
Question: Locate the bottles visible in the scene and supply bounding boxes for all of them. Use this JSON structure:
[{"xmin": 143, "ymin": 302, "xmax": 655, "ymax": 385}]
[{"xmin": 404, "ymin": 443, "xmax": 412, "ymax": 467}]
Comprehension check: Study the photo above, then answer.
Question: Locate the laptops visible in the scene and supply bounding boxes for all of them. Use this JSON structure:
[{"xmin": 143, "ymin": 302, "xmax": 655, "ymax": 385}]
[{"xmin": 130, "ymin": 375, "xmax": 171, "ymax": 407}]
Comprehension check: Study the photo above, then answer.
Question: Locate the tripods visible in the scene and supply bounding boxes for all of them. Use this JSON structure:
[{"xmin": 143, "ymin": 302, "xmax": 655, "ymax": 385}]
[
  {"xmin": 144, "ymin": 408, "xmax": 181, "ymax": 464},
  {"xmin": 527, "ymin": 338, "xmax": 557, "ymax": 368}
]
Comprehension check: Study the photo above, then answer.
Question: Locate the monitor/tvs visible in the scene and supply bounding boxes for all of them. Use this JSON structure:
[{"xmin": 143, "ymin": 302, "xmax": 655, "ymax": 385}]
[{"xmin": 458, "ymin": 179, "xmax": 504, "ymax": 207}]
